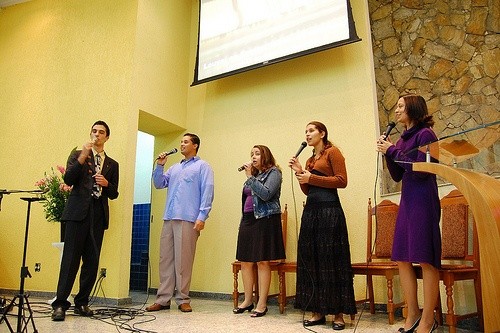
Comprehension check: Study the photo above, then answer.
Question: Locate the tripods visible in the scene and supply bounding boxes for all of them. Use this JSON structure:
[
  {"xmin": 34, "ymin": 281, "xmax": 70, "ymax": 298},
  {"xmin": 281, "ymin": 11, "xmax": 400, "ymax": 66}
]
[{"xmin": 0, "ymin": 202, "xmax": 39, "ymax": 333}]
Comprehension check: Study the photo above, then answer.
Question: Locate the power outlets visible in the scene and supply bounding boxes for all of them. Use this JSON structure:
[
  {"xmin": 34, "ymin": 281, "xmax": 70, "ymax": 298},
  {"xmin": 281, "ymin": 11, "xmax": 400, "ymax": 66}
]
[
  {"xmin": 100, "ymin": 268, "xmax": 107, "ymax": 278},
  {"xmin": 35, "ymin": 262, "xmax": 41, "ymax": 272}
]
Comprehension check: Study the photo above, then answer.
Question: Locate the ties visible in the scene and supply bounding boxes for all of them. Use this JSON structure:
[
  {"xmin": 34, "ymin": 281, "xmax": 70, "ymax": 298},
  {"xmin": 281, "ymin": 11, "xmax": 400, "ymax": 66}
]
[{"xmin": 93, "ymin": 154, "xmax": 102, "ymax": 197}]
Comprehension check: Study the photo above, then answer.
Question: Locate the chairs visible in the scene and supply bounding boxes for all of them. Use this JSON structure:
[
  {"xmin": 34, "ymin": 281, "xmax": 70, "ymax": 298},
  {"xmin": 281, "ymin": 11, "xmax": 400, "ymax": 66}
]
[
  {"xmin": 412, "ymin": 188, "xmax": 485, "ymax": 333},
  {"xmin": 349, "ymin": 197, "xmax": 408, "ymax": 324},
  {"xmin": 230, "ymin": 203, "xmax": 288, "ymax": 316},
  {"xmin": 278, "ymin": 201, "xmax": 305, "ymax": 316}
]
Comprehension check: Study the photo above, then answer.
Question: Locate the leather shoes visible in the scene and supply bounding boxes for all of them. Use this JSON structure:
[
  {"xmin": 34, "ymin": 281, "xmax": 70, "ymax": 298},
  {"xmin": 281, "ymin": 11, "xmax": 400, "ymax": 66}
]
[
  {"xmin": 304, "ymin": 316, "xmax": 325, "ymax": 326},
  {"xmin": 52, "ymin": 305, "xmax": 65, "ymax": 320},
  {"xmin": 74, "ymin": 304, "xmax": 93, "ymax": 316},
  {"xmin": 145, "ymin": 303, "xmax": 170, "ymax": 311},
  {"xmin": 332, "ymin": 321, "xmax": 345, "ymax": 330},
  {"xmin": 178, "ymin": 303, "xmax": 192, "ymax": 312}
]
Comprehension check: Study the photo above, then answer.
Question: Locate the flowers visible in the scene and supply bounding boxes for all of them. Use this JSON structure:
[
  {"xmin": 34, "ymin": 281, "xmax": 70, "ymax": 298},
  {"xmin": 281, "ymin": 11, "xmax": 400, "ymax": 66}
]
[{"xmin": 32, "ymin": 145, "xmax": 78, "ymax": 222}]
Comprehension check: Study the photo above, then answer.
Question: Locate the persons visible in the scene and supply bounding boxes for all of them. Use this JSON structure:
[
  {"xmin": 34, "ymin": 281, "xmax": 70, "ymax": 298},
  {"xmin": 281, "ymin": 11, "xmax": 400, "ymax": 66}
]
[
  {"xmin": 233, "ymin": 145, "xmax": 286, "ymax": 317},
  {"xmin": 376, "ymin": 93, "xmax": 441, "ymax": 333},
  {"xmin": 51, "ymin": 120, "xmax": 119, "ymax": 321},
  {"xmin": 289, "ymin": 122, "xmax": 357, "ymax": 330},
  {"xmin": 145, "ymin": 133, "xmax": 214, "ymax": 311}
]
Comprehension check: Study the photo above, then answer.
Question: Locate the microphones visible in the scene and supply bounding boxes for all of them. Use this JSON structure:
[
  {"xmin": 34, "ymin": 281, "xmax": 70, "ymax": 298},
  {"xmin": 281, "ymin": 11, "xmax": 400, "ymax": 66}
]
[
  {"xmin": 92, "ymin": 138, "xmax": 98, "ymax": 143},
  {"xmin": 294, "ymin": 142, "xmax": 307, "ymax": 157},
  {"xmin": 237, "ymin": 161, "xmax": 252, "ymax": 171},
  {"xmin": 156, "ymin": 148, "xmax": 178, "ymax": 160},
  {"xmin": 384, "ymin": 121, "xmax": 396, "ymax": 141}
]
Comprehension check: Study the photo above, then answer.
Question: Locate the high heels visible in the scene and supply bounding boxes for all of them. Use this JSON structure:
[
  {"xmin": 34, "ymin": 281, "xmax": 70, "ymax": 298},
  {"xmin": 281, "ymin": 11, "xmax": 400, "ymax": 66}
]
[
  {"xmin": 251, "ymin": 307, "xmax": 268, "ymax": 317},
  {"xmin": 416, "ymin": 319, "xmax": 438, "ymax": 333},
  {"xmin": 398, "ymin": 317, "xmax": 421, "ymax": 333},
  {"xmin": 233, "ymin": 303, "xmax": 254, "ymax": 313}
]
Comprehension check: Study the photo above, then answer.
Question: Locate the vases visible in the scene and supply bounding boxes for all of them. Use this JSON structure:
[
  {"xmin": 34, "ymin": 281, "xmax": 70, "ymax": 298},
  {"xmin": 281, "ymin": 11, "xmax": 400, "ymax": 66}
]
[{"xmin": 60, "ymin": 219, "xmax": 65, "ymax": 243}]
